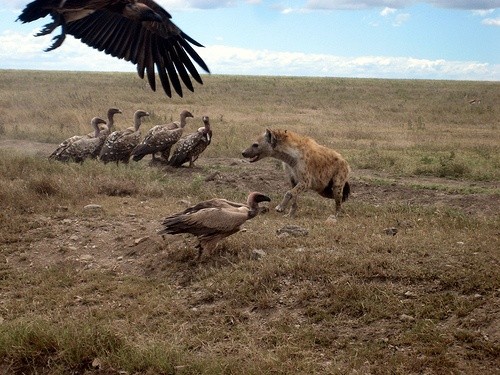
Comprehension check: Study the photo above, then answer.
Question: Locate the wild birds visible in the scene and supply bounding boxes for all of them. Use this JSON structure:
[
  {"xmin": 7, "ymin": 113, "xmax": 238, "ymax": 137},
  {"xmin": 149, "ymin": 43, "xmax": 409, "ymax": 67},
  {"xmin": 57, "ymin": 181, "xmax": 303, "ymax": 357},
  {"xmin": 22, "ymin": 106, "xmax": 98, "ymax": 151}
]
[
  {"xmin": 162, "ymin": 190, "xmax": 270, "ymax": 264},
  {"xmin": 47, "ymin": 107, "xmax": 212, "ymax": 171}
]
